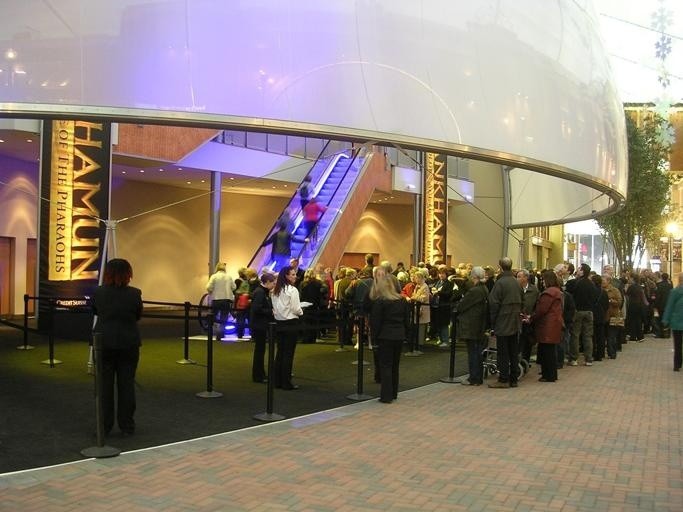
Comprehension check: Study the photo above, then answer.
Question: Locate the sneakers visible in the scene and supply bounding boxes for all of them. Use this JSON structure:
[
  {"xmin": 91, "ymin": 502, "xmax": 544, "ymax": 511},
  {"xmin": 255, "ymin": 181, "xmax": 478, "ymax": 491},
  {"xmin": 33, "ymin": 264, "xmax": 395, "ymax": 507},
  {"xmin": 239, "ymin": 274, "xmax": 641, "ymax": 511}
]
[
  {"xmin": 567, "ymin": 360, "xmax": 578, "ymax": 366},
  {"xmin": 585, "ymin": 362, "xmax": 593, "ymax": 366}
]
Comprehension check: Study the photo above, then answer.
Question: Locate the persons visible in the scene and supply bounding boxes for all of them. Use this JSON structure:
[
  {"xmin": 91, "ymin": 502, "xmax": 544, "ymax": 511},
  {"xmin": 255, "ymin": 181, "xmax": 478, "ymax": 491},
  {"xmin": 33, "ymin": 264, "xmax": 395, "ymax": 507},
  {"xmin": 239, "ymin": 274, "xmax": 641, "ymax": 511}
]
[
  {"xmin": 364, "ymin": 261, "xmax": 412, "ymax": 403},
  {"xmin": 302, "ymin": 198, "xmax": 327, "ymax": 242},
  {"xmin": 274, "ymin": 209, "xmax": 296, "ymax": 235},
  {"xmin": 259, "ymin": 222, "xmax": 310, "ymax": 272},
  {"xmin": 232, "ymin": 258, "xmax": 304, "ymax": 392},
  {"xmin": 589, "ymin": 265, "xmax": 674, "ymax": 361},
  {"xmin": 90, "ymin": 259, "xmax": 143, "ymax": 437},
  {"xmin": 662, "ymin": 272, "xmax": 683, "ymax": 372},
  {"xmin": 393, "ymin": 262, "xmax": 475, "ymax": 347},
  {"xmin": 295, "ymin": 175, "xmax": 315, "ymax": 210},
  {"xmin": 455, "ymin": 257, "xmax": 598, "ymax": 389},
  {"xmin": 206, "ymin": 263, "xmax": 237, "ymax": 341},
  {"xmin": 300, "ymin": 253, "xmax": 377, "ymax": 352}
]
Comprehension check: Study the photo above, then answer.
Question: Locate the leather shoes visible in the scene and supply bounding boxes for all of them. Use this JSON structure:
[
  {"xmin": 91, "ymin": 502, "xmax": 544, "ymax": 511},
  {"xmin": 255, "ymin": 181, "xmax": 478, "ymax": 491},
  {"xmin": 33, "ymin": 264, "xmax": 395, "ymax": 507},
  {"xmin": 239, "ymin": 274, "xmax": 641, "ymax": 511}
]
[
  {"xmin": 538, "ymin": 377, "xmax": 554, "ymax": 383},
  {"xmin": 488, "ymin": 382, "xmax": 510, "ymax": 389},
  {"xmin": 510, "ymin": 382, "xmax": 518, "ymax": 387},
  {"xmin": 461, "ymin": 378, "xmax": 480, "ymax": 386}
]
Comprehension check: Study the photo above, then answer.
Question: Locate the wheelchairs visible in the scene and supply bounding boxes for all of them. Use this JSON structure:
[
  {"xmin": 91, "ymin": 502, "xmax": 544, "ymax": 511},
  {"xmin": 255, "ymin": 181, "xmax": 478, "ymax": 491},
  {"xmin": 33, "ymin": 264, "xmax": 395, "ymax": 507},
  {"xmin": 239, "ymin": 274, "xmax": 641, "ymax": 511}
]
[{"xmin": 480, "ymin": 325, "xmax": 531, "ymax": 381}]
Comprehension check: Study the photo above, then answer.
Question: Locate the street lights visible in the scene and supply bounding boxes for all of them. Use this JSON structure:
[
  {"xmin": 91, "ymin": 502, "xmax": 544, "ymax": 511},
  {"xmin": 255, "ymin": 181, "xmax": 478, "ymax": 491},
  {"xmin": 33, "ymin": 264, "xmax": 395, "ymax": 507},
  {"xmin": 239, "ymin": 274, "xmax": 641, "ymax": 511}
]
[{"xmin": 664, "ymin": 221, "xmax": 679, "ymax": 283}]
